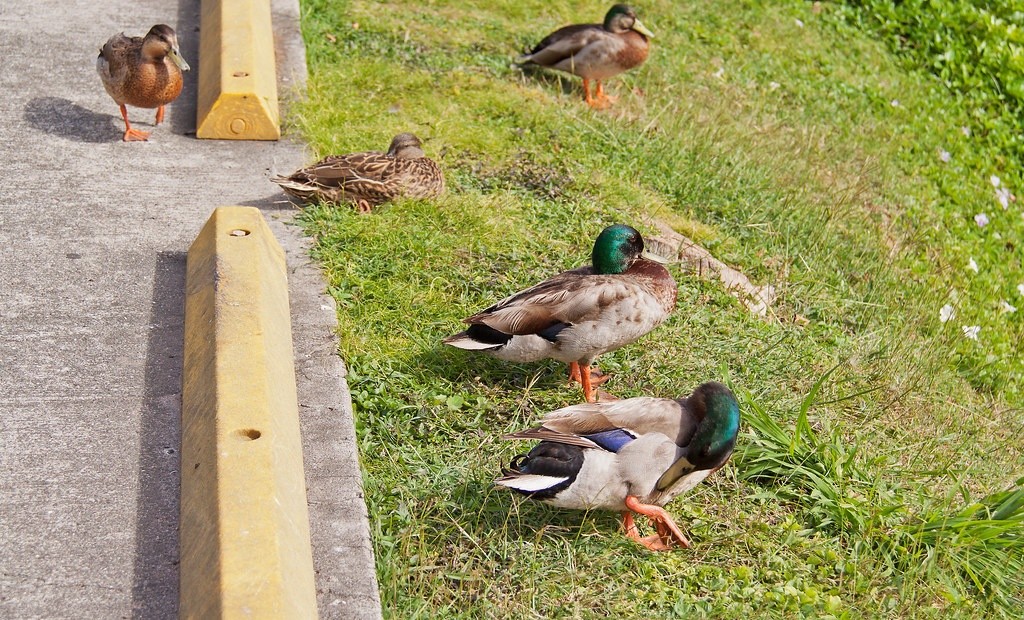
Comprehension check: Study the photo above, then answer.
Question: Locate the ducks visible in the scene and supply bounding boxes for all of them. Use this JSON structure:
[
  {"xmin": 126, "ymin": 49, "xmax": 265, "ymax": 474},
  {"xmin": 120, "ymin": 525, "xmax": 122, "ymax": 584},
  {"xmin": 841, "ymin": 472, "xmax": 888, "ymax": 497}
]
[
  {"xmin": 97, "ymin": 24, "xmax": 190, "ymax": 142},
  {"xmin": 270, "ymin": 133, "xmax": 444, "ymax": 213}
]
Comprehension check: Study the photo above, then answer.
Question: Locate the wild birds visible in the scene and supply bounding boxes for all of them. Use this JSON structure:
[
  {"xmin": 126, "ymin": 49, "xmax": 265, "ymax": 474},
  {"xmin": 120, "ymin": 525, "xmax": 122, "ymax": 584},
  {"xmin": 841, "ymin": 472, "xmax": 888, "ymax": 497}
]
[
  {"xmin": 441, "ymin": 226, "xmax": 677, "ymax": 402},
  {"xmin": 513, "ymin": 4, "xmax": 650, "ymax": 108},
  {"xmin": 492, "ymin": 382, "xmax": 739, "ymax": 555}
]
